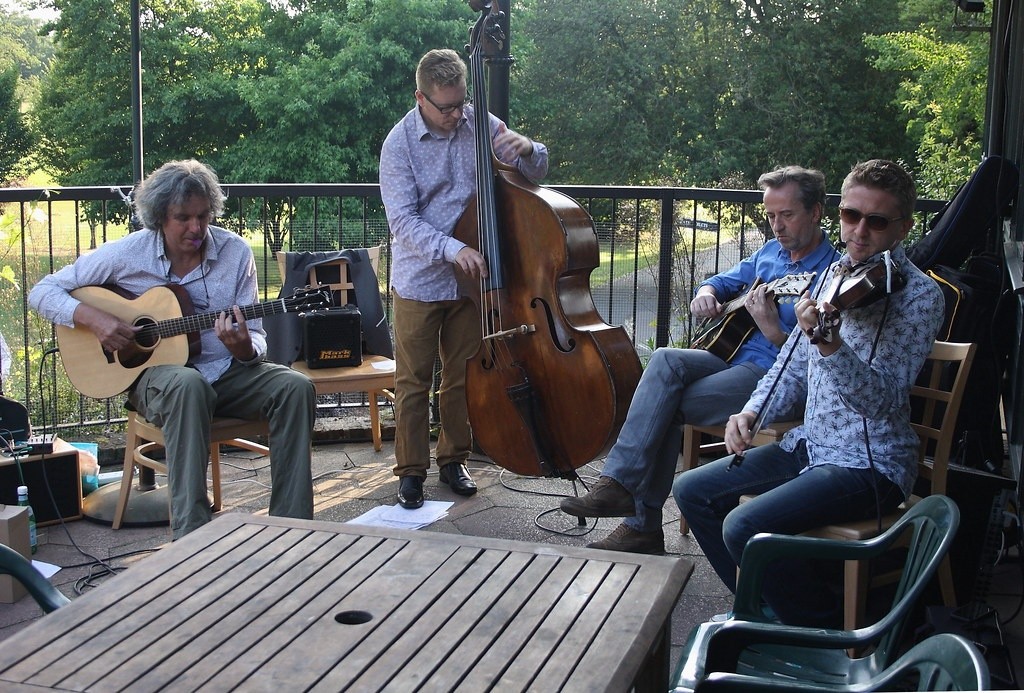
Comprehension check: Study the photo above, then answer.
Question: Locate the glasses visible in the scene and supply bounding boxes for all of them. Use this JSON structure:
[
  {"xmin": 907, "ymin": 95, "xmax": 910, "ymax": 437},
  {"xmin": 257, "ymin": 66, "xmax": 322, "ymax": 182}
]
[
  {"xmin": 420, "ymin": 91, "xmax": 474, "ymax": 115},
  {"xmin": 840, "ymin": 207, "xmax": 902, "ymax": 231}
]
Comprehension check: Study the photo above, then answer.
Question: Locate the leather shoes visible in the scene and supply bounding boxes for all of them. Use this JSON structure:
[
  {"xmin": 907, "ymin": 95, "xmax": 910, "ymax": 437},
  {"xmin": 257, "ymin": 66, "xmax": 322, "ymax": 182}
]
[
  {"xmin": 439, "ymin": 462, "xmax": 478, "ymax": 496},
  {"xmin": 397, "ymin": 476, "xmax": 424, "ymax": 509}
]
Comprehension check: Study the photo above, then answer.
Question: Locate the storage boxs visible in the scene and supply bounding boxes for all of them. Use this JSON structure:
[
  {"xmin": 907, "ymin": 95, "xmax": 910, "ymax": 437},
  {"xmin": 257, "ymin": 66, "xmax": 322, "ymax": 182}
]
[{"xmin": 0, "ymin": 504, "xmax": 32, "ymax": 604}]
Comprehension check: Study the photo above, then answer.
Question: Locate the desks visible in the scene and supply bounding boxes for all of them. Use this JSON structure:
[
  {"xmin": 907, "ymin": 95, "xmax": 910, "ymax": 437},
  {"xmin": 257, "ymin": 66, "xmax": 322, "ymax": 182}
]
[{"xmin": 0, "ymin": 511, "xmax": 696, "ymax": 693}]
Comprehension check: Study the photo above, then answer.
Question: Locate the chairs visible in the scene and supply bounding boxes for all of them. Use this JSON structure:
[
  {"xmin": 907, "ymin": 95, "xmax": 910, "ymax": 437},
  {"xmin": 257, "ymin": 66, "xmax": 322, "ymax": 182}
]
[
  {"xmin": 276, "ymin": 246, "xmax": 396, "ymax": 453},
  {"xmin": 736, "ymin": 339, "xmax": 978, "ymax": 658},
  {"xmin": 669, "ymin": 494, "xmax": 961, "ymax": 693},
  {"xmin": 112, "ymin": 408, "xmax": 269, "ymax": 530},
  {"xmin": 681, "ymin": 419, "xmax": 804, "ymax": 535},
  {"xmin": 694, "ymin": 633, "xmax": 991, "ymax": 693}
]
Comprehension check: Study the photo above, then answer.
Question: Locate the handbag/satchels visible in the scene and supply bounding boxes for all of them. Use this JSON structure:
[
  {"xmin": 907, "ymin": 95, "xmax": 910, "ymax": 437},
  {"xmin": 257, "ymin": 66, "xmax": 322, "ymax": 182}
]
[{"xmin": 909, "ymin": 257, "xmax": 1013, "ymax": 432}]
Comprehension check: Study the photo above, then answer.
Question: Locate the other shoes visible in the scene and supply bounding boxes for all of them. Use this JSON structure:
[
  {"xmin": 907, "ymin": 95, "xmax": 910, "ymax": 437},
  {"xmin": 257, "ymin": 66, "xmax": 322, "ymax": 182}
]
[{"xmin": 710, "ymin": 605, "xmax": 781, "ymax": 624}]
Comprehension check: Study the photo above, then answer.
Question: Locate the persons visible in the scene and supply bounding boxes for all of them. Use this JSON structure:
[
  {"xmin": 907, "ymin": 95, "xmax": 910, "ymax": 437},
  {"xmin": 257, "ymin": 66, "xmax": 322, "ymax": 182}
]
[
  {"xmin": 379, "ymin": 48, "xmax": 549, "ymax": 509},
  {"xmin": 26, "ymin": 158, "xmax": 318, "ymax": 543},
  {"xmin": 560, "ymin": 165, "xmax": 842, "ymax": 556},
  {"xmin": 671, "ymin": 158, "xmax": 949, "ymax": 629}
]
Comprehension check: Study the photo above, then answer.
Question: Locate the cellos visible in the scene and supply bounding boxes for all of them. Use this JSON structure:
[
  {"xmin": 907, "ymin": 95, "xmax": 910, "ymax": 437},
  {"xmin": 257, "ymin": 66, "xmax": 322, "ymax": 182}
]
[{"xmin": 451, "ymin": 0, "xmax": 644, "ymax": 527}]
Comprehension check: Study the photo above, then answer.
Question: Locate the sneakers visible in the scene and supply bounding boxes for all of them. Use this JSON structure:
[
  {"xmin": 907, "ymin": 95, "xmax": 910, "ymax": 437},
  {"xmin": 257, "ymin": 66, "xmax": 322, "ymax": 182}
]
[
  {"xmin": 561, "ymin": 477, "xmax": 636, "ymax": 517},
  {"xmin": 586, "ymin": 523, "xmax": 665, "ymax": 555}
]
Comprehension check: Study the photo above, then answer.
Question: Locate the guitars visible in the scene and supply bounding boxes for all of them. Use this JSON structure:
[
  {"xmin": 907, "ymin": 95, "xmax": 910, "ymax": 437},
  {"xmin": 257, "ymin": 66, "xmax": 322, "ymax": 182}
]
[
  {"xmin": 688, "ymin": 270, "xmax": 818, "ymax": 362},
  {"xmin": 55, "ymin": 282, "xmax": 336, "ymax": 398}
]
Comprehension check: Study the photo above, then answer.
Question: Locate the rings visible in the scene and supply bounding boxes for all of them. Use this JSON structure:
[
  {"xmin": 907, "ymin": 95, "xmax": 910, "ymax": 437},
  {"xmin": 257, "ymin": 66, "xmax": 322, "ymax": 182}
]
[{"xmin": 748, "ymin": 303, "xmax": 754, "ymax": 307}]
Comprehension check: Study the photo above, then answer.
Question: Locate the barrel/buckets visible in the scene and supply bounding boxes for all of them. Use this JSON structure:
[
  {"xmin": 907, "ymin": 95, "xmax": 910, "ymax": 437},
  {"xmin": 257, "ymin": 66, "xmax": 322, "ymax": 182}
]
[{"xmin": 69, "ymin": 442, "xmax": 98, "ymax": 498}]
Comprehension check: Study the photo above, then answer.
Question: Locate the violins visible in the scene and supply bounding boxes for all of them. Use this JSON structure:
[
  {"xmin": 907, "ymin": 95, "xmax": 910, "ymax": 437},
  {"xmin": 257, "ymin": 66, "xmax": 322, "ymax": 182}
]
[{"xmin": 805, "ymin": 250, "xmax": 901, "ymax": 346}]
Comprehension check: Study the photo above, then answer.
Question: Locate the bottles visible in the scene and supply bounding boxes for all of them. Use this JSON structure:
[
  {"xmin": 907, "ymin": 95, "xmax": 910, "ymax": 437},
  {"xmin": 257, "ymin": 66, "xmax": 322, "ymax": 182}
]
[{"xmin": 17, "ymin": 486, "xmax": 37, "ymax": 554}]
